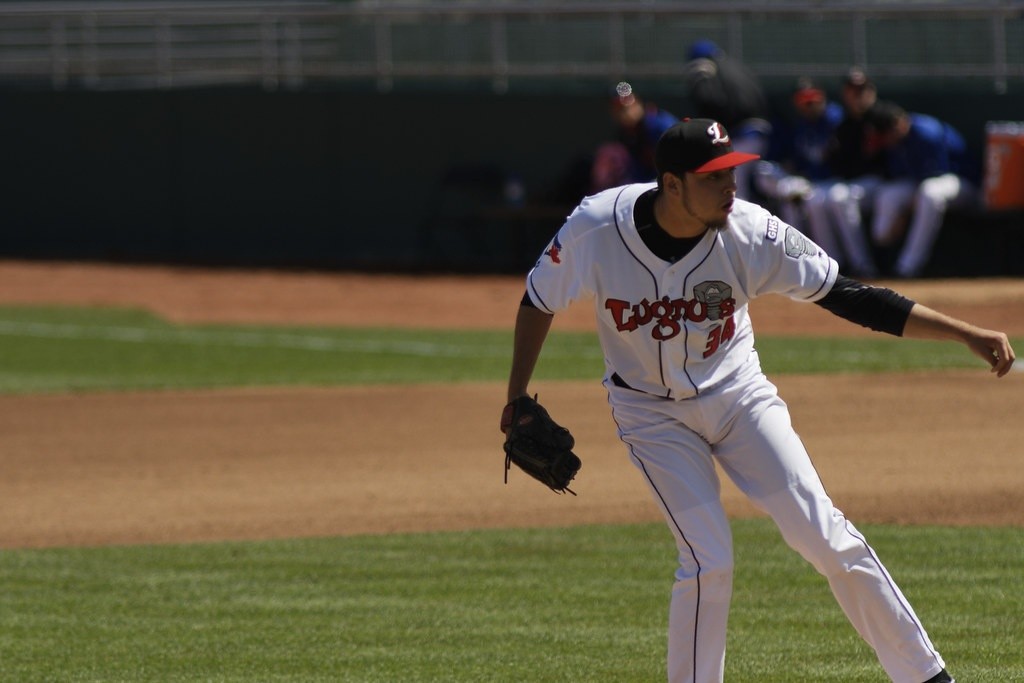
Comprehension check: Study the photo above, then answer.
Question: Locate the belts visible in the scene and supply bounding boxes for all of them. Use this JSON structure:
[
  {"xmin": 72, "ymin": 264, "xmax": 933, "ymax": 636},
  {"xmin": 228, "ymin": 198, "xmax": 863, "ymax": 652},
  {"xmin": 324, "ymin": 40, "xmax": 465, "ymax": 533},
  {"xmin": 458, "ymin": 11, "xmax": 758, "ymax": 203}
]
[{"xmin": 610, "ymin": 373, "xmax": 674, "ymax": 401}]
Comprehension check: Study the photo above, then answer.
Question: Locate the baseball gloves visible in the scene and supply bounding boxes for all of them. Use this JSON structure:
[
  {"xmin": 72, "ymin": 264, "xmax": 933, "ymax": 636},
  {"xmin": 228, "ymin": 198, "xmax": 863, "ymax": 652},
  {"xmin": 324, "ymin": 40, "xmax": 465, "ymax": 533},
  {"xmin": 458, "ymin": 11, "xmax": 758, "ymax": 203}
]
[{"xmin": 500, "ymin": 394, "xmax": 583, "ymax": 491}]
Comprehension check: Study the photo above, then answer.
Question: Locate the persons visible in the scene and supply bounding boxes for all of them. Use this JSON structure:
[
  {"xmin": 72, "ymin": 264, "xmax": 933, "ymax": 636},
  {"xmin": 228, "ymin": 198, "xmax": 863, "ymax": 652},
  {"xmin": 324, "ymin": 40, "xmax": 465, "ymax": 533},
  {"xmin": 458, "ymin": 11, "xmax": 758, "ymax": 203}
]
[
  {"xmin": 589, "ymin": 40, "xmax": 987, "ymax": 283},
  {"xmin": 499, "ymin": 116, "xmax": 1018, "ymax": 683}
]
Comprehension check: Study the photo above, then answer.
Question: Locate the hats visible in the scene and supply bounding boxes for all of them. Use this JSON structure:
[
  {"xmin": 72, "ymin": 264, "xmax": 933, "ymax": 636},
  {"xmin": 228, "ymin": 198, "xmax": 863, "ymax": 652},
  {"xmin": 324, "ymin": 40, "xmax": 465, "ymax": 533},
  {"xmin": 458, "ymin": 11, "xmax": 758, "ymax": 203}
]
[
  {"xmin": 609, "ymin": 93, "xmax": 634, "ymax": 114},
  {"xmin": 686, "ymin": 57, "xmax": 715, "ymax": 82},
  {"xmin": 871, "ymin": 101, "xmax": 902, "ymax": 133},
  {"xmin": 840, "ymin": 69, "xmax": 876, "ymax": 92},
  {"xmin": 794, "ymin": 78, "xmax": 824, "ymax": 107},
  {"xmin": 654, "ymin": 117, "xmax": 761, "ymax": 178}
]
